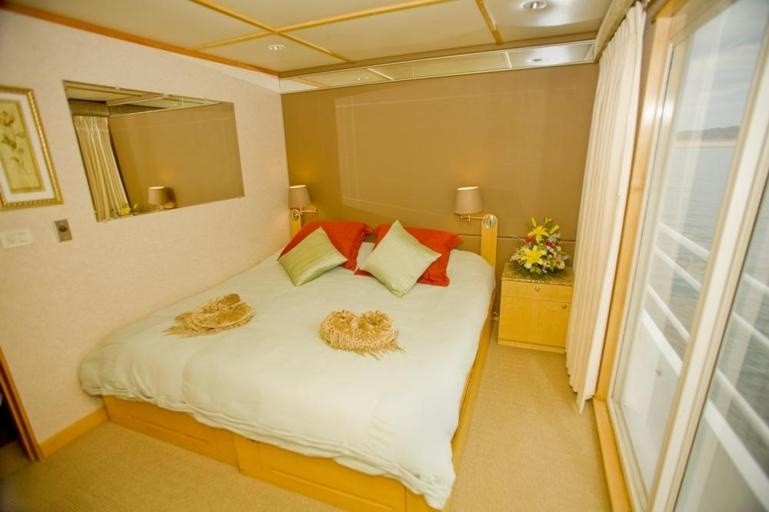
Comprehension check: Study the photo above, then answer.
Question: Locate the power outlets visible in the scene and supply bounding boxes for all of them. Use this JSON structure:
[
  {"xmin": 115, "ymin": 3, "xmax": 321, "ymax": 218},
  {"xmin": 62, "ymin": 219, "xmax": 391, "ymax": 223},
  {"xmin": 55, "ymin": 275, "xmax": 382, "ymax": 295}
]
[{"xmin": 51, "ymin": 218, "xmax": 73, "ymax": 242}]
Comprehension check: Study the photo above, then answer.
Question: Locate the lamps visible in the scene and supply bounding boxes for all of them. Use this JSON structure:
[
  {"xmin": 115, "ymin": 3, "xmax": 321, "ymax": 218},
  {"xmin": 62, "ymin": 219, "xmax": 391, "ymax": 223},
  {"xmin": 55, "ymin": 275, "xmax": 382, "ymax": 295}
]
[
  {"xmin": 148, "ymin": 186, "xmax": 171, "ymax": 211},
  {"xmin": 287, "ymin": 184, "xmax": 311, "ymax": 221},
  {"xmin": 454, "ymin": 186, "xmax": 496, "ymax": 228}
]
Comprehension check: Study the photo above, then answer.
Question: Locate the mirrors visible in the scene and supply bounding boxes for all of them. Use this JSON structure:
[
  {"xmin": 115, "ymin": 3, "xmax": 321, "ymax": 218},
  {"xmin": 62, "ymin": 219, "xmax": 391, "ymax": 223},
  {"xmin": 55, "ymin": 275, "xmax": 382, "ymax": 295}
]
[{"xmin": 62, "ymin": 79, "xmax": 245, "ymax": 223}]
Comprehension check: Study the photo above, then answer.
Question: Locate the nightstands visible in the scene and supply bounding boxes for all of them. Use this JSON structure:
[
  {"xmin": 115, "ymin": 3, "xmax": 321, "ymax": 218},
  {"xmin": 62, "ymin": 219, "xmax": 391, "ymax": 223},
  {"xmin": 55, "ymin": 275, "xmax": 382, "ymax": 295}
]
[{"xmin": 498, "ymin": 261, "xmax": 575, "ymax": 354}]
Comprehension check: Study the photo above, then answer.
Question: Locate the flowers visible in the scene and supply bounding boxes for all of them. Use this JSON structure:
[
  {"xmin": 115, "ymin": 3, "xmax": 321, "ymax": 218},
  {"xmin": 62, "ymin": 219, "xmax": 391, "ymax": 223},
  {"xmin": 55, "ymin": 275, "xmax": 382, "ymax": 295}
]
[{"xmin": 508, "ymin": 216, "xmax": 571, "ymax": 281}]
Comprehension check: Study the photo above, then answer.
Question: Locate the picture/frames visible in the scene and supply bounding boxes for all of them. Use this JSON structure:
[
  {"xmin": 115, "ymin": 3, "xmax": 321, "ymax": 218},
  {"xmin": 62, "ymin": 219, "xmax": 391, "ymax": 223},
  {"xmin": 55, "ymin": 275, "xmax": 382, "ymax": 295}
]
[{"xmin": 0, "ymin": 85, "xmax": 64, "ymax": 212}]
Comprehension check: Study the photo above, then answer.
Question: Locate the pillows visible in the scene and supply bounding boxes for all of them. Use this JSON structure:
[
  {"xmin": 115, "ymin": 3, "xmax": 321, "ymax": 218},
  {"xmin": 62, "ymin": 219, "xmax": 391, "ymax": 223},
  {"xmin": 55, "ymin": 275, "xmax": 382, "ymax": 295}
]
[
  {"xmin": 277, "ymin": 220, "xmax": 374, "ymax": 270},
  {"xmin": 359, "ymin": 219, "xmax": 442, "ymax": 296},
  {"xmin": 279, "ymin": 226, "xmax": 348, "ymax": 286},
  {"xmin": 354, "ymin": 224, "xmax": 463, "ymax": 287}
]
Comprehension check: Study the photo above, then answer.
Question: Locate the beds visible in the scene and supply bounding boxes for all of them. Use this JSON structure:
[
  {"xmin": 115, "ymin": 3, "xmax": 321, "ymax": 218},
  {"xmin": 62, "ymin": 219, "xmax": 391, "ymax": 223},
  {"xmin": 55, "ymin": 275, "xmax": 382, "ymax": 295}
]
[{"xmin": 78, "ymin": 209, "xmax": 498, "ymax": 511}]
